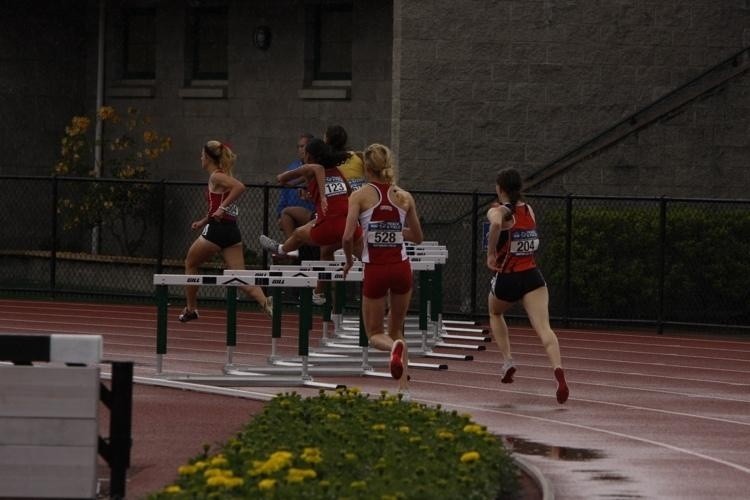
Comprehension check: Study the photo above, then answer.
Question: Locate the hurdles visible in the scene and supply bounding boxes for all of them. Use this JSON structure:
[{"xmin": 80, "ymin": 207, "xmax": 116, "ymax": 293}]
[
  {"xmin": 0, "ymin": 333, "xmax": 133, "ymax": 500},
  {"xmin": 153, "ymin": 242, "xmax": 490, "ymax": 394}
]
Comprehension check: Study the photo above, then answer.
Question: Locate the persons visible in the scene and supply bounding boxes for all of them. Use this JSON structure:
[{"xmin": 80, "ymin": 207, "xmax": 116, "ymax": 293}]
[
  {"xmin": 275, "ymin": 134, "xmax": 321, "ymax": 303},
  {"xmin": 256, "ymin": 140, "xmax": 363, "ymax": 263},
  {"xmin": 341, "ymin": 142, "xmax": 425, "ymax": 399},
  {"xmin": 176, "ymin": 141, "xmax": 272, "ymax": 323},
  {"xmin": 486, "ymin": 170, "xmax": 570, "ymax": 404},
  {"xmin": 324, "ymin": 125, "xmax": 363, "ymax": 195}
]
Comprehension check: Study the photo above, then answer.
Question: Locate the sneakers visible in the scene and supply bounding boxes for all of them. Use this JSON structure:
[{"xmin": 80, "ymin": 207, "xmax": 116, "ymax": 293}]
[
  {"xmin": 554, "ymin": 365, "xmax": 569, "ymax": 404},
  {"xmin": 263, "ymin": 296, "xmax": 273, "ymax": 320},
  {"xmin": 259, "ymin": 235, "xmax": 286, "ymax": 256},
  {"xmin": 178, "ymin": 307, "xmax": 199, "ymax": 322},
  {"xmin": 501, "ymin": 359, "xmax": 516, "ymax": 383},
  {"xmin": 390, "ymin": 340, "xmax": 406, "ymax": 381},
  {"xmin": 297, "ymin": 289, "xmax": 326, "ymax": 308}
]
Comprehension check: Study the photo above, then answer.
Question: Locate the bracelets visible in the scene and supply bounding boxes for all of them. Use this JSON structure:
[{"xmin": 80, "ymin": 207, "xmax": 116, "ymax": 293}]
[{"xmin": 218, "ymin": 205, "xmax": 229, "ymax": 212}]
